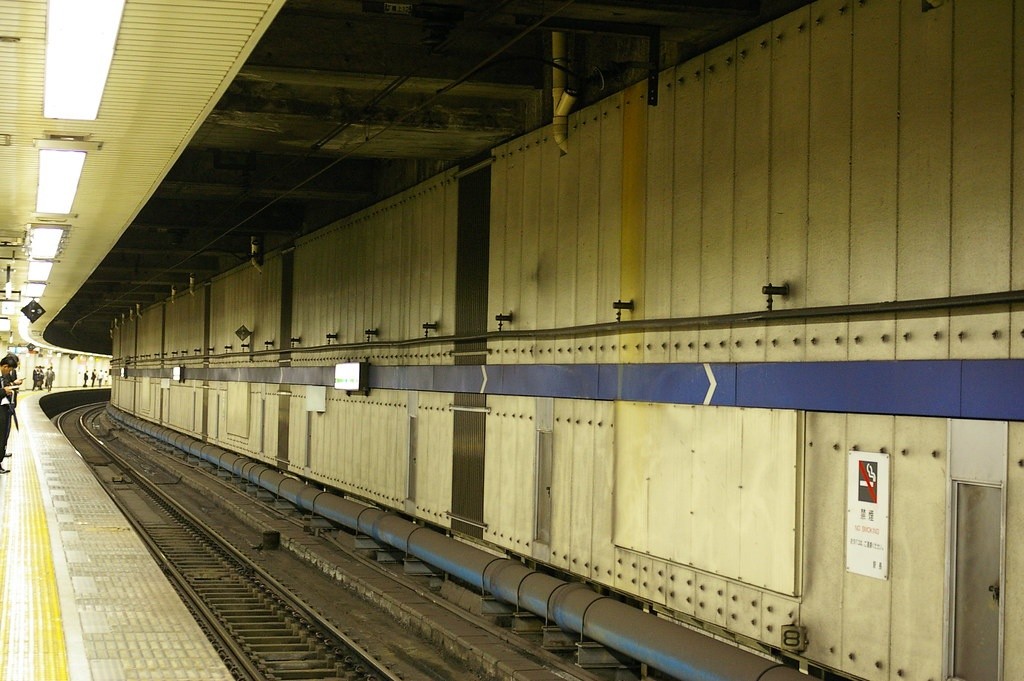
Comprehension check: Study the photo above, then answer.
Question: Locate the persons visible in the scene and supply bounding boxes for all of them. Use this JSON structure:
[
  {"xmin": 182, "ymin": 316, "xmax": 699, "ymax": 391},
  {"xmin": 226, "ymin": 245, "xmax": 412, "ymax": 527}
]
[
  {"xmin": 32, "ymin": 366, "xmax": 55, "ymax": 392},
  {"xmin": 91, "ymin": 369, "xmax": 108, "ymax": 387},
  {"xmin": 0, "ymin": 353, "xmax": 23, "ymax": 473},
  {"xmin": 83, "ymin": 371, "xmax": 88, "ymax": 386}
]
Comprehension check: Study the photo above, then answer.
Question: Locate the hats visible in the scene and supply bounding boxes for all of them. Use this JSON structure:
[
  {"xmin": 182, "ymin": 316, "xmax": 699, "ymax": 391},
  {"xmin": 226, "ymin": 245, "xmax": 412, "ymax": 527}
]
[
  {"xmin": 35, "ymin": 366, "xmax": 39, "ymax": 368},
  {"xmin": 38, "ymin": 366, "xmax": 44, "ymax": 368}
]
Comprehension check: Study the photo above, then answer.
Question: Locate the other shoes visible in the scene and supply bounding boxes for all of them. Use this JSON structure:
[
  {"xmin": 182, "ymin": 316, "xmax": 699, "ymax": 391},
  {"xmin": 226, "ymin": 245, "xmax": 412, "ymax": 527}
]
[
  {"xmin": 4, "ymin": 452, "xmax": 13, "ymax": 457},
  {"xmin": 0, "ymin": 468, "xmax": 11, "ymax": 474}
]
